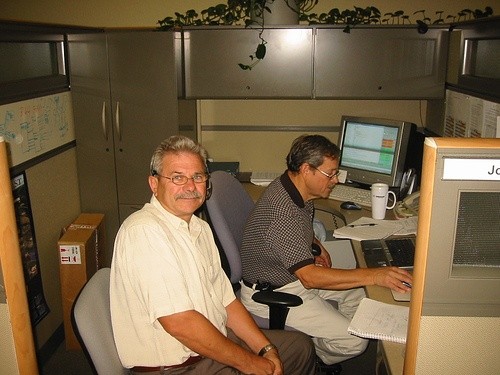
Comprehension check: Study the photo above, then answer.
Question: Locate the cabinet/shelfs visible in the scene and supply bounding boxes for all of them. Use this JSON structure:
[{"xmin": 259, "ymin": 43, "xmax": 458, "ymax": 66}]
[
  {"xmin": 67, "ymin": 32, "xmax": 197, "ymax": 267},
  {"xmin": 183, "ymin": 28, "xmax": 314, "ymax": 101},
  {"xmin": 314, "ymin": 28, "xmax": 450, "ymax": 100}
]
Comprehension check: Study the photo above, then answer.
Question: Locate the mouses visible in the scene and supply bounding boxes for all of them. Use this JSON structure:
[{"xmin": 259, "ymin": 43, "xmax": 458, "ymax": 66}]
[
  {"xmin": 401, "ymin": 281, "xmax": 412, "ymax": 288},
  {"xmin": 340, "ymin": 201, "xmax": 362, "ymax": 210}
]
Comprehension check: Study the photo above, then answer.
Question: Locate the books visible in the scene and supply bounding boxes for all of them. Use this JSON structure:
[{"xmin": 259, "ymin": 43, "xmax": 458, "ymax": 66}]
[{"xmin": 347, "ymin": 298, "xmax": 410, "ymax": 344}]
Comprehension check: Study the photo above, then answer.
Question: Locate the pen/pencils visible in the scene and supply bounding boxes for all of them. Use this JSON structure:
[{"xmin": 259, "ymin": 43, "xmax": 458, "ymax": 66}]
[{"xmin": 351, "ymin": 224, "xmax": 377, "ymax": 227}]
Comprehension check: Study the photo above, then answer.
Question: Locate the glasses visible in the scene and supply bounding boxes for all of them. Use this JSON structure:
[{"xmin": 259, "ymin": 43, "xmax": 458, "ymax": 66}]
[
  {"xmin": 298, "ymin": 160, "xmax": 343, "ymax": 178},
  {"xmin": 151, "ymin": 170, "xmax": 210, "ymax": 186}
]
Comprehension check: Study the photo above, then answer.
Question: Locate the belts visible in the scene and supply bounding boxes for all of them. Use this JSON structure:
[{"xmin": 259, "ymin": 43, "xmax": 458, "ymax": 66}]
[
  {"xmin": 242, "ymin": 278, "xmax": 279, "ymax": 290},
  {"xmin": 130, "ymin": 355, "xmax": 203, "ymax": 373}
]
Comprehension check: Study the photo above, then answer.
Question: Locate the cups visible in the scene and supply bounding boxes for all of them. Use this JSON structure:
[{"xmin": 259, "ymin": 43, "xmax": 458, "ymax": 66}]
[{"xmin": 371, "ymin": 183, "xmax": 396, "ymax": 220}]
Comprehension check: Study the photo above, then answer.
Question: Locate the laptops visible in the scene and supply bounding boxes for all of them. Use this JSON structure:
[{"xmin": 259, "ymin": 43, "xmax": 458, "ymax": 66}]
[{"xmin": 360, "ymin": 237, "xmax": 416, "ymax": 269}]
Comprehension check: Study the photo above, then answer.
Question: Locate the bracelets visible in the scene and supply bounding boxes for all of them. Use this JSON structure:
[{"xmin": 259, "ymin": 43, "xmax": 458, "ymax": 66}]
[{"xmin": 257, "ymin": 343, "xmax": 276, "ymax": 357}]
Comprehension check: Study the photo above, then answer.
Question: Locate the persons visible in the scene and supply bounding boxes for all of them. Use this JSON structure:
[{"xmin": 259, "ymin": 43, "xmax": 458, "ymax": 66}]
[
  {"xmin": 241, "ymin": 135, "xmax": 414, "ymax": 375},
  {"xmin": 109, "ymin": 134, "xmax": 316, "ymax": 375}
]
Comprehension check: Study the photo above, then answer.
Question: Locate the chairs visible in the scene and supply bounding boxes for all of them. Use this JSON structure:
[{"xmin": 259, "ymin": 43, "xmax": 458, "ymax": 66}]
[
  {"xmin": 206, "ymin": 170, "xmax": 342, "ymax": 375},
  {"xmin": 74, "ymin": 268, "xmax": 128, "ymax": 375}
]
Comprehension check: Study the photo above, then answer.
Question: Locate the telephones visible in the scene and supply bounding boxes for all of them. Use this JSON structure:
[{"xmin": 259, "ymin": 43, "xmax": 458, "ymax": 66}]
[{"xmin": 402, "ymin": 191, "xmax": 420, "ymax": 215}]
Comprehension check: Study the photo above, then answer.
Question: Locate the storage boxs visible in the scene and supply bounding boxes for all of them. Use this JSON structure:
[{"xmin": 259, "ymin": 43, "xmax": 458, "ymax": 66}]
[{"xmin": 57, "ymin": 214, "xmax": 106, "ymax": 351}]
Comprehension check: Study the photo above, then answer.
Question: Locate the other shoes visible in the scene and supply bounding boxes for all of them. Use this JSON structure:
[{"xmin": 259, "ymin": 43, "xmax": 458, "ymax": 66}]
[{"xmin": 315, "ymin": 355, "xmax": 342, "ymax": 375}]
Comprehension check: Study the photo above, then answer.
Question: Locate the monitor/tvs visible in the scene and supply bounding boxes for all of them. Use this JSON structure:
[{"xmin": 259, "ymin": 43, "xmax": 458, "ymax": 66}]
[{"xmin": 337, "ymin": 115, "xmax": 435, "ymax": 191}]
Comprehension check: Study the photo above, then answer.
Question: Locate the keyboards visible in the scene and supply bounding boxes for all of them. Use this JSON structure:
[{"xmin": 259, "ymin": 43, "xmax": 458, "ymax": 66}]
[{"xmin": 328, "ymin": 185, "xmax": 372, "ymax": 208}]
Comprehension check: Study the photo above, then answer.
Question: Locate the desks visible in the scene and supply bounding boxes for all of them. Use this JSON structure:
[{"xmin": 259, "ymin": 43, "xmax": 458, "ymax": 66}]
[{"xmin": 232, "ymin": 182, "xmax": 416, "ymax": 375}]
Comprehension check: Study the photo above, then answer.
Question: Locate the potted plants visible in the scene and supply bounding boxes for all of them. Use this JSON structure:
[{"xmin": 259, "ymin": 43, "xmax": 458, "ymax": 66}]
[{"xmin": 151, "ymin": 0, "xmax": 494, "ymax": 71}]
